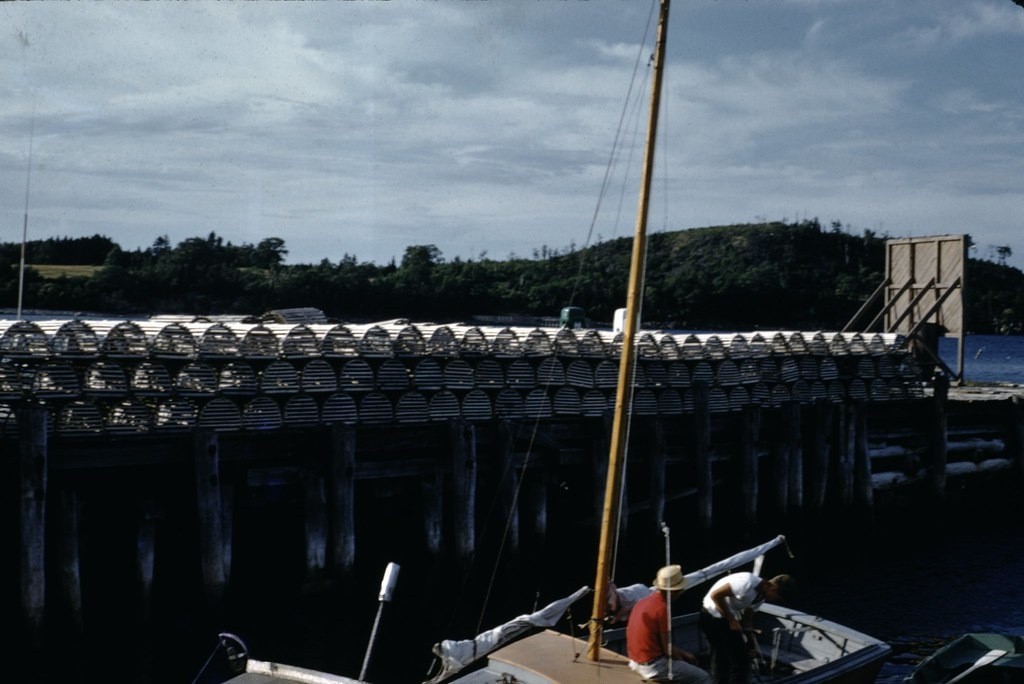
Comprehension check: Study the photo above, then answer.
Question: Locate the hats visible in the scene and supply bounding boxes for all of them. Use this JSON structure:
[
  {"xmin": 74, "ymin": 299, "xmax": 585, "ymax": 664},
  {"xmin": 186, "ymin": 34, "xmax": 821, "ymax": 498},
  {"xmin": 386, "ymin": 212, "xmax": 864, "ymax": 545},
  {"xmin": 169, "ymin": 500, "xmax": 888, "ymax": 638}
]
[{"xmin": 652, "ymin": 565, "xmax": 687, "ymax": 590}]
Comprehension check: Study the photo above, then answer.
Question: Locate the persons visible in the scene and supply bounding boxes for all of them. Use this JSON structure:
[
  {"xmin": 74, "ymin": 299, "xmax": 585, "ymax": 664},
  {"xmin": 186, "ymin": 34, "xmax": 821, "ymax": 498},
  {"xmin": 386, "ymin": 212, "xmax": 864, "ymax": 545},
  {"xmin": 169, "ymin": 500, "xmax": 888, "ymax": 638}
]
[
  {"xmin": 698, "ymin": 573, "xmax": 794, "ymax": 684},
  {"xmin": 626, "ymin": 565, "xmax": 711, "ymax": 684}
]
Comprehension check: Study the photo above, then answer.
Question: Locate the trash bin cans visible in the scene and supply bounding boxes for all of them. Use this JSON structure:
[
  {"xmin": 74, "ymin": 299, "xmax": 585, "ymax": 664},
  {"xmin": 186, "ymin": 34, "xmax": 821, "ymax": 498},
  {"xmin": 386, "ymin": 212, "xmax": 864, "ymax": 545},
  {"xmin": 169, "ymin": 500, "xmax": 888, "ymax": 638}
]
[
  {"xmin": 560, "ymin": 307, "xmax": 587, "ymax": 330},
  {"xmin": 612, "ymin": 308, "xmax": 628, "ymax": 334}
]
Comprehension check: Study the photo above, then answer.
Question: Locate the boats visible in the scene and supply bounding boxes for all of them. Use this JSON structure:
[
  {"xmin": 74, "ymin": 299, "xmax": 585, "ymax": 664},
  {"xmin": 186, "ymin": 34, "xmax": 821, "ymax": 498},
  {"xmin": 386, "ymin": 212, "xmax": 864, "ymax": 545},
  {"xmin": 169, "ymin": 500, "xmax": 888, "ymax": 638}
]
[
  {"xmin": 904, "ymin": 633, "xmax": 1015, "ymax": 684},
  {"xmin": 184, "ymin": 2, "xmax": 892, "ymax": 684}
]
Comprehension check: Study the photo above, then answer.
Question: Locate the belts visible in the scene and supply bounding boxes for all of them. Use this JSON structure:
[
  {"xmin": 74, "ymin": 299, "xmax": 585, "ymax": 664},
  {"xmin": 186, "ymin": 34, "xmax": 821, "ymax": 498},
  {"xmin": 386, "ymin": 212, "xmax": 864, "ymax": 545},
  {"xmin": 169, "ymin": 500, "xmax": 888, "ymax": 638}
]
[{"xmin": 641, "ymin": 654, "xmax": 663, "ymax": 665}]
